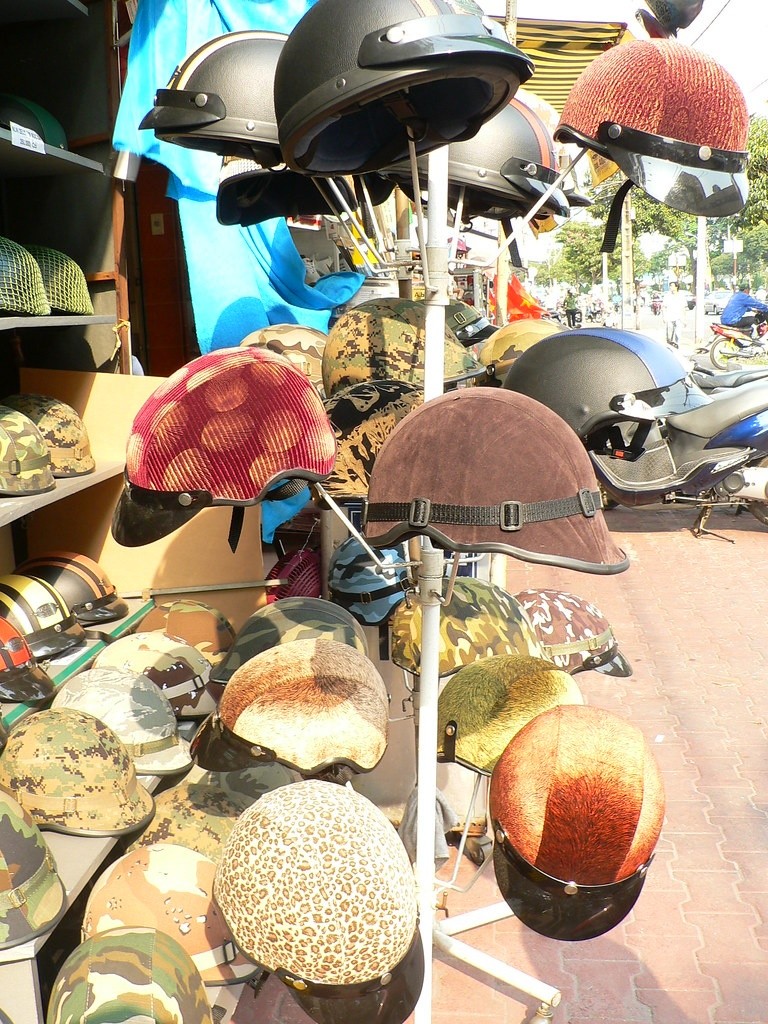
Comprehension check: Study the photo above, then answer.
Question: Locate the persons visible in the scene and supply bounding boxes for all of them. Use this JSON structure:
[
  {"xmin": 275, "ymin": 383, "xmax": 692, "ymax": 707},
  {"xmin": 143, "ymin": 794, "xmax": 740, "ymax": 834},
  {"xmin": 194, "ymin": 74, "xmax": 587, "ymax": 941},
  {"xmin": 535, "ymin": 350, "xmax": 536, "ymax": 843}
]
[
  {"xmin": 563, "ymin": 290, "xmax": 577, "ymax": 328},
  {"xmin": 721, "ymin": 284, "xmax": 768, "ymax": 356},
  {"xmin": 652, "ymin": 292, "xmax": 663, "ymax": 312},
  {"xmin": 612, "ymin": 293, "xmax": 622, "ymax": 311},
  {"xmin": 662, "ymin": 282, "xmax": 688, "ymax": 346}
]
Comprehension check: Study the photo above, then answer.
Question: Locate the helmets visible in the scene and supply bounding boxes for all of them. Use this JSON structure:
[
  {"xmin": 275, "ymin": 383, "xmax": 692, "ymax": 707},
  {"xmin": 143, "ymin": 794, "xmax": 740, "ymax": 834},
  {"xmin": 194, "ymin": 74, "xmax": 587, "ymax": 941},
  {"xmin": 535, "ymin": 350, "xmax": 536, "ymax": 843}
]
[
  {"xmin": 0, "ymin": 0, "xmax": 666, "ymax": 1024},
  {"xmin": 554, "ymin": 37, "xmax": 751, "ymax": 217},
  {"xmin": 360, "ymin": 386, "xmax": 630, "ymax": 575},
  {"xmin": 504, "ymin": 327, "xmax": 715, "ymax": 463}
]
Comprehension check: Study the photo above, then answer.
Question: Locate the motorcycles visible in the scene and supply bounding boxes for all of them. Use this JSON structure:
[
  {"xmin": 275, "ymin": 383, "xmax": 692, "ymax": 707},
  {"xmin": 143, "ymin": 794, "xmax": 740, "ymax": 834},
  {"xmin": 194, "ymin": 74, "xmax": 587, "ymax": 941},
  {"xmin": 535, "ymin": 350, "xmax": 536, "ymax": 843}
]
[{"xmin": 709, "ymin": 311, "xmax": 768, "ymax": 370}]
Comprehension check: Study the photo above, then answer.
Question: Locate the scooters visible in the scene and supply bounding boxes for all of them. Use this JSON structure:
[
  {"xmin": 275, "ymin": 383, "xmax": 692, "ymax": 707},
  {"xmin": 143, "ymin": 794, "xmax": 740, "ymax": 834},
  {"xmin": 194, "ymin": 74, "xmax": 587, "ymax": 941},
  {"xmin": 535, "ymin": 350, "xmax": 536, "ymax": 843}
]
[{"xmin": 598, "ymin": 322, "xmax": 768, "ymax": 544}]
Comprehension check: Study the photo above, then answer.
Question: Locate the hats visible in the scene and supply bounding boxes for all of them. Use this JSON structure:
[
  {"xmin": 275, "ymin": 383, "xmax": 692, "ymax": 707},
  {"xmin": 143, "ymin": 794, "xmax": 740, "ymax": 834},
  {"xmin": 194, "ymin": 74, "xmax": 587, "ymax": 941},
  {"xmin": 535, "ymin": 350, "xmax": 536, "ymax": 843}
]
[{"xmin": 740, "ymin": 283, "xmax": 751, "ymax": 291}]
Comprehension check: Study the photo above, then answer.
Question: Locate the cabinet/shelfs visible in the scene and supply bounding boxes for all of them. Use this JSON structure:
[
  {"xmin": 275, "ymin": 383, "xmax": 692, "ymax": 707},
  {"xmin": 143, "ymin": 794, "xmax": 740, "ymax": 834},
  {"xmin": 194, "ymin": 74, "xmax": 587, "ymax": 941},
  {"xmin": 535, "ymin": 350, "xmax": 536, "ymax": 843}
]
[{"xmin": 0, "ymin": 2, "xmax": 245, "ymax": 1023}]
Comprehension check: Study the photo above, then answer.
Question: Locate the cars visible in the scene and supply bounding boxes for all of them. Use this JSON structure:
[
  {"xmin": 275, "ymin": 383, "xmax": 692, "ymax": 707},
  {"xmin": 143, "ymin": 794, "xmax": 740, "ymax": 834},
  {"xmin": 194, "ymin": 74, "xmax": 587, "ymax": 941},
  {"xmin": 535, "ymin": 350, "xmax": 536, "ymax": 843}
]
[
  {"xmin": 632, "ymin": 288, "xmax": 696, "ymax": 316},
  {"xmin": 704, "ymin": 290, "xmax": 734, "ymax": 315}
]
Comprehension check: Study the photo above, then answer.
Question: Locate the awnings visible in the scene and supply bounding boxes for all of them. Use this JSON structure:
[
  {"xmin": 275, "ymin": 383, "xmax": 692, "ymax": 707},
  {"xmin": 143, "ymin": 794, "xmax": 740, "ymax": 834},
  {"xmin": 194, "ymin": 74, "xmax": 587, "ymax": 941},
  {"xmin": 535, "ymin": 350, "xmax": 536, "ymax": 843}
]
[{"xmin": 488, "ymin": 15, "xmax": 655, "ymax": 236}]
